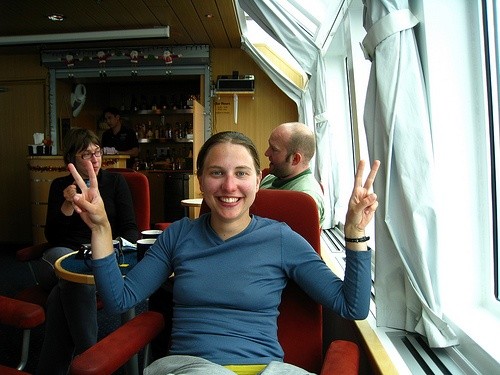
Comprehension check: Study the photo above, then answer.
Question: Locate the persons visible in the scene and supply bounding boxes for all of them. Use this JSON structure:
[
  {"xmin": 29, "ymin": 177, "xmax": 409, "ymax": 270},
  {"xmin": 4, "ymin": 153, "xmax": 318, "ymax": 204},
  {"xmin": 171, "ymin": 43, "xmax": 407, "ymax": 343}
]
[
  {"xmin": 47, "ymin": 131, "xmax": 138, "ymax": 375},
  {"xmin": 100, "ymin": 110, "xmax": 138, "ymax": 151},
  {"xmin": 65, "ymin": 131, "xmax": 381, "ymax": 375},
  {"xmin": 258, "ymin": 123, "xmax": 326, "ymax": 236}
]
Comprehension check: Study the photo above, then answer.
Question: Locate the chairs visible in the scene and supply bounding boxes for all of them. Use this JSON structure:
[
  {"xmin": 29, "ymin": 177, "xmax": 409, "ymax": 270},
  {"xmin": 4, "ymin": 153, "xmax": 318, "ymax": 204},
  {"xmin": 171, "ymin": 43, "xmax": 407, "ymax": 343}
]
[
  {"xmin": 106, "ymin": 169, "xmax": 150, "ymax": 239},
  {"xmin": 0, "ymin": 295, "xmax": 46, "ymax": 375},
  {"xmin": 70, "ymin": 190, "xmax": 361, "ymax": 375}
]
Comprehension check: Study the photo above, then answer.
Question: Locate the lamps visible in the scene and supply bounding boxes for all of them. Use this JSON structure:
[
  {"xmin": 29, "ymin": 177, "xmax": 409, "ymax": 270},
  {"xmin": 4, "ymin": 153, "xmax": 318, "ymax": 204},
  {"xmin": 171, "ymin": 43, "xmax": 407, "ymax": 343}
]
[{"xmin": 0, "ymin": 26, "xmax": 171, "ymax": 45}]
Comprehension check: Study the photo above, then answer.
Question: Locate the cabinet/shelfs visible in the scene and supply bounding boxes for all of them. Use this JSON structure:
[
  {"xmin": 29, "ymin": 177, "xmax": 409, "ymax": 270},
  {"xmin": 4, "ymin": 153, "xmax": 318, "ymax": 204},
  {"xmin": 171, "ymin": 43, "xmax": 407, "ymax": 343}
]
[{"xmin": 104, "ymin": 103, "xmax": 195, "ymax": 225}]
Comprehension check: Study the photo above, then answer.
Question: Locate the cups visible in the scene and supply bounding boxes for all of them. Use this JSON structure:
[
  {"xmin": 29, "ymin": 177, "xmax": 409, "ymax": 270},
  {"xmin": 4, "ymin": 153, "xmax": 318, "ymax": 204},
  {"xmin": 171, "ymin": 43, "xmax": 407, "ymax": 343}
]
[
  {"xmin": 76, "ymin": 178, "xmax": 90, "ymax": 194},
  {"xmin": 79, "ymin": 244, "xmax": 92, "ymax": 259},
  {"xmin": 141, "ymin": 230, "xmax": 163, "ymax": 239},
  {"xmin": 112, "ymin": 240, "xmax": 122, "ymax": 259},
  {"xmin": 137, "ymin": 239, "xmax": 157, "ymax": 260}
]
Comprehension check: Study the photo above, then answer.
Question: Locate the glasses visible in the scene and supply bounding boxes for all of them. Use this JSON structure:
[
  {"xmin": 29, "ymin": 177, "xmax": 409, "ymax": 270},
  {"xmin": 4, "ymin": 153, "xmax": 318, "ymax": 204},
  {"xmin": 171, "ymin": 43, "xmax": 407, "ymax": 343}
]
[{"xmin": 70, "ymin": 149, "xmax": 104, "ymax": 160}]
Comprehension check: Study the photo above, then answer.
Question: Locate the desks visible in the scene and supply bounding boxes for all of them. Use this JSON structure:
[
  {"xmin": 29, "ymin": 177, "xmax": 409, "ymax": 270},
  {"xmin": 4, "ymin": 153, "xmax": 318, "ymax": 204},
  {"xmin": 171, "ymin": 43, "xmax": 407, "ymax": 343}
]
[
  {"xmin": 54, "ymin": 243, "xmax": 175, "ymax": 375},
  {"xmin": 181, "ymin": 197, "xmax": 204, "ymax": 220}
]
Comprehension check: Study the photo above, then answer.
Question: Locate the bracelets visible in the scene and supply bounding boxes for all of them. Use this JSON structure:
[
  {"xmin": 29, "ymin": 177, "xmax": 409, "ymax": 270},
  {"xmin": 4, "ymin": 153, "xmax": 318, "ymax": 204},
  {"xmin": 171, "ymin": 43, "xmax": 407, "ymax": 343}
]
[
  {"xmin": 65, "ymin": 198, "xmax": 72, "ymax": 208},
  {"xmin": 344, "ymin": 236, "xmax": 370, "ymax": 243}
]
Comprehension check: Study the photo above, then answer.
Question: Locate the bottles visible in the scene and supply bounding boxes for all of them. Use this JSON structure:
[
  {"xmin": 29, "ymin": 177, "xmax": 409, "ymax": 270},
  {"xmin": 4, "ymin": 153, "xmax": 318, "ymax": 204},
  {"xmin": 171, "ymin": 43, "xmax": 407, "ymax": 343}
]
[{"xmin": 98, "ymin": 94, "xmax": 200, "ymax": 171}]
